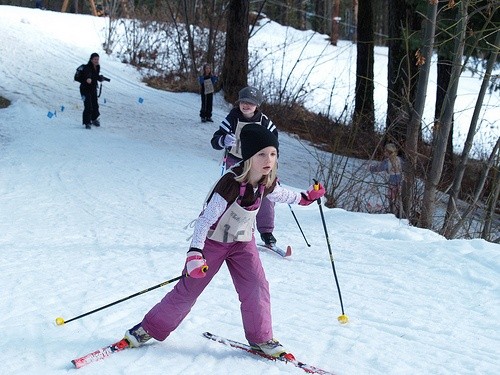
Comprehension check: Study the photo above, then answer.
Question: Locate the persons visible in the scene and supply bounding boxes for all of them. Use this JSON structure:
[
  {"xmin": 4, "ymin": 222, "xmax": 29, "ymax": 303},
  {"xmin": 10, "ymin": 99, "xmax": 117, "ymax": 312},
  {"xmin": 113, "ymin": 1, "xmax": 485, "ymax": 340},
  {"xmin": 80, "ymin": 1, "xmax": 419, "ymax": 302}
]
[
  {"xmin": 199, "ymin": 63, "xmax": 218, "ymax": 123},
  {"xmin": 211, "ymin": 86, "xmax": 280, "ymax": 244},
  {"xmin": 371, "ymin": 143, "xmax": 403, "ymax": 201},
  {"xmin": 74, "ymin": 53, "xmax": 104, "ymax": 129},
  {"xmin": 125, "ymin": 123, "xmax": 325, "ymax": 357}
]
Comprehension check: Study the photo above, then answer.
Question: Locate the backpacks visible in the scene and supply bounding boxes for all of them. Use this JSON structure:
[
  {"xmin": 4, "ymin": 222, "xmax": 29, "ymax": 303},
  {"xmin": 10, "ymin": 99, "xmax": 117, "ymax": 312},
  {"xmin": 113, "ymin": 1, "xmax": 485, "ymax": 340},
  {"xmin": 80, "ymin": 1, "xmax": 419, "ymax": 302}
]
[{"xmin": 74, "ymin": 64, "xmax": 87, "ymax": 83}]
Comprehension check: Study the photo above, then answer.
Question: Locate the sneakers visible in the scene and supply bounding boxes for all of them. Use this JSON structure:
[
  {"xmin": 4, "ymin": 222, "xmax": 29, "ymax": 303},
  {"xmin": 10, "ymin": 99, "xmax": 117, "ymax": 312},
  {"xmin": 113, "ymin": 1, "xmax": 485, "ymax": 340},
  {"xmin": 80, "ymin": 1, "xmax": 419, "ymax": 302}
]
[
  {"xmin": 249, "ymin": 339, "xmax": 287, "ymax": 358},
  {"xmin": 125, "ymin": 322, "xmax": 152, "ymax": 348}
]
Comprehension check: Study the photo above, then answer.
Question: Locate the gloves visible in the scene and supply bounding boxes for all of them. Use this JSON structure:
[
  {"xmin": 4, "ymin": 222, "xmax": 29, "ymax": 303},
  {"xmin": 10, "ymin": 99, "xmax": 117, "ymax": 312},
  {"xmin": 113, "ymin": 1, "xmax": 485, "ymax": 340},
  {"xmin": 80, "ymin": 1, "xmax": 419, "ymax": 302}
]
[
  {"xmin": 298, "ymin": 182, "xmax": 326, "ymax": 206},
  {"xmin": 185, "ymin": 251, "xmax": 208, "ymax": 279},
  {"xmin": 224, "ymin": 134, "xmax": 236, "ymax": 147}
]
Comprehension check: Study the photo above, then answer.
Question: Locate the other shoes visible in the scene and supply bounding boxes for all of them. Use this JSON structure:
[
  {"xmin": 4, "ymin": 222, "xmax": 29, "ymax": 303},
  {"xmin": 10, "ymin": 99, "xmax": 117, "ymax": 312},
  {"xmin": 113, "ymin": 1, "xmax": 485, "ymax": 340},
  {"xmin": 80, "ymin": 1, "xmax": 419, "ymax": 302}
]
[
  {"xmin": 93, "ymin": 120, "xmax": 100, "ymax": 126},
  {"xmin": 86, "ymin": 123, "xmax": 91, "ymax": 130},
  {"xmin": 261, "ymin": 233, "xmax": 276, "ymax": 244},
  {"xmin": 201, "ymin": 117, "xmax": 206, "ymax": 122},
  {"xmin": 207, "ymin": 117, "xmax": 213, "ymax": 122}
]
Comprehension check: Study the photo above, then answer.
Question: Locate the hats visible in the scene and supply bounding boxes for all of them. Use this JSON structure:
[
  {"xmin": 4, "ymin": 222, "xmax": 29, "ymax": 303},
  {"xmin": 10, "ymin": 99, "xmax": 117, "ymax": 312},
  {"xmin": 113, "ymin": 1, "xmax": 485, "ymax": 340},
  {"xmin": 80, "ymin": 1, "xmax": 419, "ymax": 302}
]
[
  {"xmin": 235, "ymin": 86, "xmax": 263, "ymax": 106},
  {"xmin": 240, "ymin": 123, "xmax": 279, "ymax": 162}
]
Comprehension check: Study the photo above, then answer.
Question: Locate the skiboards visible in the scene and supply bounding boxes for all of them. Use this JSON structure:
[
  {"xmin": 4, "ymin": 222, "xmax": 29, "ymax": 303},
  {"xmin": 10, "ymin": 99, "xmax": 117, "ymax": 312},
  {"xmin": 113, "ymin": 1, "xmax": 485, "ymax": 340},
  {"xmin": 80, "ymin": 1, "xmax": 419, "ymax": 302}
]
[
  {"xmin": 256, "ymin": 241, "xmax": 291, "ymax": 257},
  {"xmin": 70, "ymin": 332, "xmax": 339, "ymax": 375}
]
